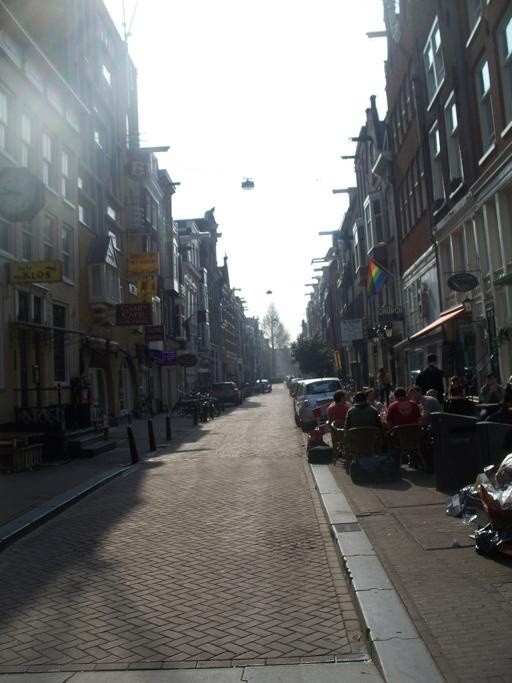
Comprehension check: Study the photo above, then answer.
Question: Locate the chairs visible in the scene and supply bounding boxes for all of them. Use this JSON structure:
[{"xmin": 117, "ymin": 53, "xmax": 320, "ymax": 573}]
[{"xmin": 326, "ymin": 390, "xmax": 512, "ymax": 478}]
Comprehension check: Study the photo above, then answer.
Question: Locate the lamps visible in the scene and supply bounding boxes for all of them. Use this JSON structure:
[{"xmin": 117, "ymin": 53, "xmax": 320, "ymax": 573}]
[{"xmin": 369, "ymin": 322, "xmax": 392, "ymax": 343}]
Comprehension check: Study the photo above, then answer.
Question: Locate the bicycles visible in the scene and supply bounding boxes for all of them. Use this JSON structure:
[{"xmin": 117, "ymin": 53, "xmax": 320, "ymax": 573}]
[{"xmin": 177, "ymin": 387, "xmax": 226, "ymax": 422}]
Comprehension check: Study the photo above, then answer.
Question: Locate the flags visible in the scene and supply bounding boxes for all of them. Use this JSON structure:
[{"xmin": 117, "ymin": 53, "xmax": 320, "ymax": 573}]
[{"xmin": 367, "ymin": 260, "xmax": 391, "ymax": 299}]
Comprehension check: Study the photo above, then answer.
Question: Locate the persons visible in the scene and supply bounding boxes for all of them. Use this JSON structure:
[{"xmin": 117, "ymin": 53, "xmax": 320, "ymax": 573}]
[
  {"xmin": 376, "ymin": 368, "xmax": 392, "ymax": 403},
  {"xmin": 448, "ymin": 375, "xmax": 465, "ymax": 409},
  {"xmin": 416, "ymin": 354, "xmax": 444, "ymax": 403},
  {"xmin": 327, "ymin": 386, "xmax": 422, "ymax": 444},
  {"xmin": 478, "ymin": 372, "xmax": 504, "ymax": 421},
  {"xmin": 425, "ymin": 389, "xmax": 448, "ymax": 412},
  {"xmin": 406, "ymin": 384, "xmax": 445, "ymax": 469}
]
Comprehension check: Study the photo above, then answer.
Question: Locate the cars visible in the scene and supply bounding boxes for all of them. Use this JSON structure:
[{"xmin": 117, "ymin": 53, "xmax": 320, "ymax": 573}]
[
  {"xmin": 210, "ymin": 379, "xmax": 242, "ymax": 408},
  {"xmin": 283, "ymin": 371, "xmax": 349, "ymax": 433},
  {"xmin": 253, "ymin": 377, "xmax": 273, "ymax": 393}
]
